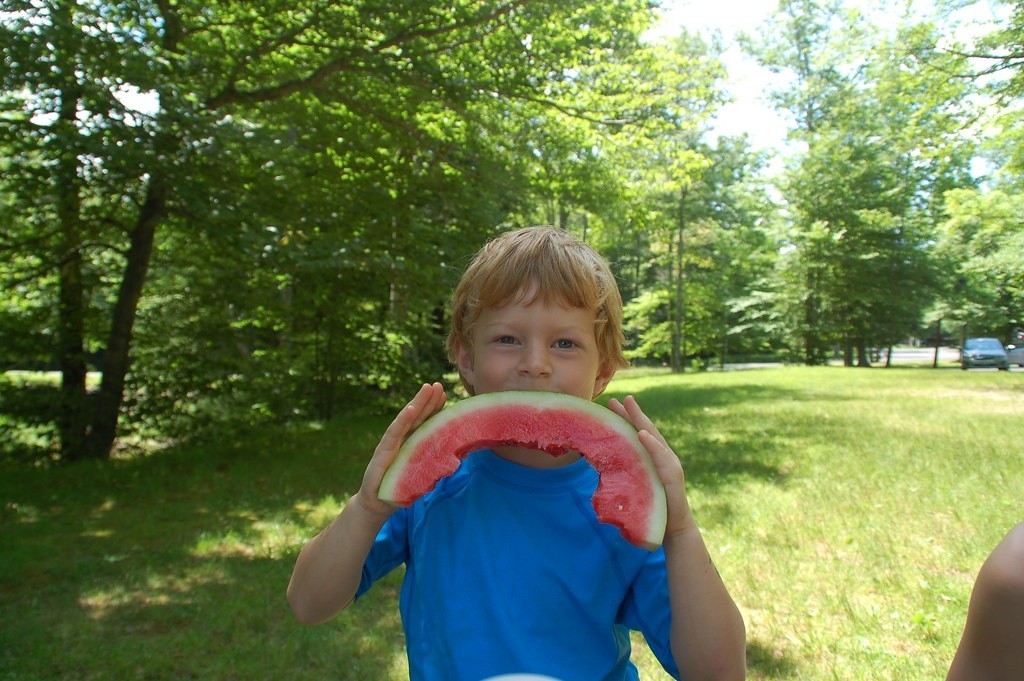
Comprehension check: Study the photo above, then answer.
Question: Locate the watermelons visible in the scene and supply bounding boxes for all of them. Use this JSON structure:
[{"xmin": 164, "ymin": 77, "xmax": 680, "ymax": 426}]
[{"xmin": 377, "ymin": 389, "xmax": 668, "ymax": 548}]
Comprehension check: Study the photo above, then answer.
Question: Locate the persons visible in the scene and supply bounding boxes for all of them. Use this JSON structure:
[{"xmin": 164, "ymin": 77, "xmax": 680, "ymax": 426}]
[
  {"xmin": 285, "ymin": 225, "xmax": 747, "ymax": 680},
  {"xmin": 945, "ymin": 520, "xmax": 1023, "ymax": 681}
]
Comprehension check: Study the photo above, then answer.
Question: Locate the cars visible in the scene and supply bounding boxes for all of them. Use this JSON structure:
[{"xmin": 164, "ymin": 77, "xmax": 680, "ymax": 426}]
[
  {"xmin": 960, "ymin": 337, "xmax": 1009, "ymax": 371},
  {"xmin": 1005, "ymin": 342, "xmax": 1024, "ymax": 366}
]
